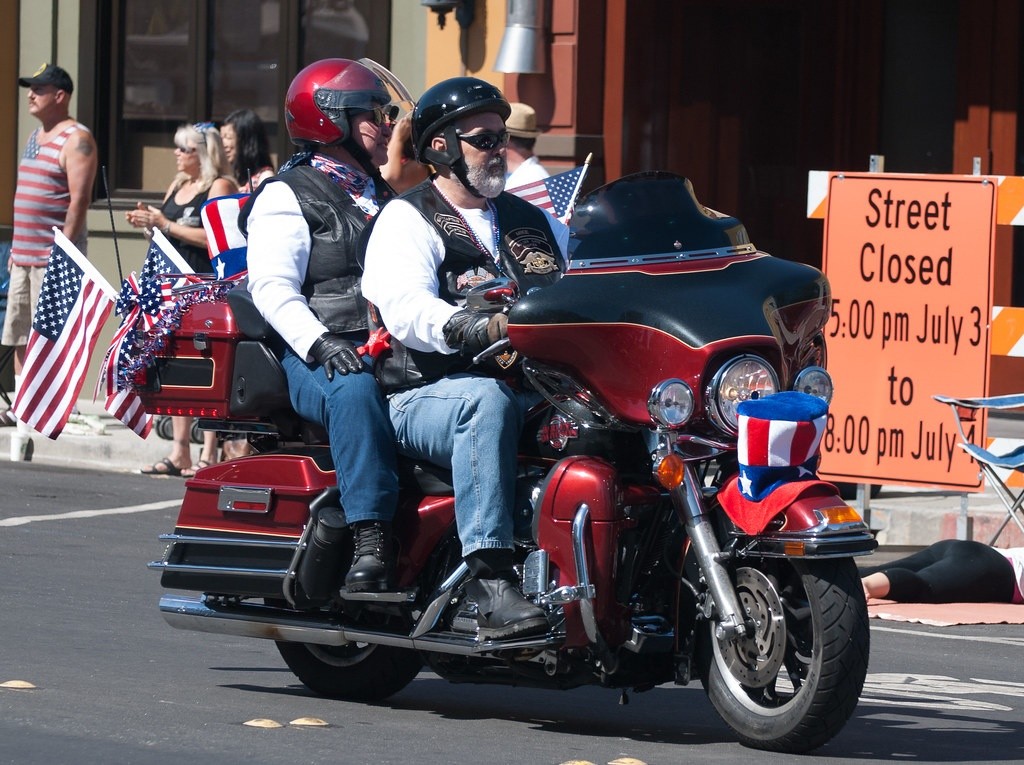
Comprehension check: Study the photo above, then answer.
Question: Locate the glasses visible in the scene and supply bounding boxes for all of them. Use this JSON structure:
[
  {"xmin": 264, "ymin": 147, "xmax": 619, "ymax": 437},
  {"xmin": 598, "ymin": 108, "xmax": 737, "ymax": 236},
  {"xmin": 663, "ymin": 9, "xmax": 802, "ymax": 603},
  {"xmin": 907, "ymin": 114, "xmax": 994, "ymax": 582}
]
[
  {"xmin": 177, "ymin": 146, "xmax": 197, "ymax": 153},
  {"xmin": 350, "ymin": 106, "xmax": 400, "ymax": 126},
  {"xmin": 435, "ymin": 131, "xmax": 511, "ymax": 151}
]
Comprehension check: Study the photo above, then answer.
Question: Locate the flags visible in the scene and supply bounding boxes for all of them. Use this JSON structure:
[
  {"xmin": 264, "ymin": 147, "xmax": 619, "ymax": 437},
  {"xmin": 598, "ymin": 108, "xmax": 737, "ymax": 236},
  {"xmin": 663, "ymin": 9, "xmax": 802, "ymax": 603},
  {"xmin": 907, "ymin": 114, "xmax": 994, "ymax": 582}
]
[
  {"xmin": 503, "ymin": 166, "xmax": 586, "ymax": 228},
  {"xmin": 103, "ymin": 234, "xmax": 199, "ymax": 440},
  {"xmin": 11, "ymin": 234, "xmax": 114, "ymax": 440}
]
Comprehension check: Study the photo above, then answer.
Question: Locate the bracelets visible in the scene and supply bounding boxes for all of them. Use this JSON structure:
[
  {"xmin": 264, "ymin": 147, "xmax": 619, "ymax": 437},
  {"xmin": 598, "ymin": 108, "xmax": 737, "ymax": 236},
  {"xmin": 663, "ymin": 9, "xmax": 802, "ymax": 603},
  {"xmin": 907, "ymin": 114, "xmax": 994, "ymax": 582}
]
[{"xmin": 162, "ymin": 222, "xmax": 170, "ymax": 235}]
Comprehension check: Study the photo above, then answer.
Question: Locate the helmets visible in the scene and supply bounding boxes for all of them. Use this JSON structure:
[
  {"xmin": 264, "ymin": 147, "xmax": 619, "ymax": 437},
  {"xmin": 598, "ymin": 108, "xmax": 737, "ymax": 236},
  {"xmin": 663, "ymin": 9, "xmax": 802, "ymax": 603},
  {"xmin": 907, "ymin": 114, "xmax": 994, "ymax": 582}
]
[
  {"xmin": 409, "ymin": 76, "xmax": 512, "ymax": 166},
  {"xmin": 283, "ymin": 58, "xmax": 392, "ymax": 147}
]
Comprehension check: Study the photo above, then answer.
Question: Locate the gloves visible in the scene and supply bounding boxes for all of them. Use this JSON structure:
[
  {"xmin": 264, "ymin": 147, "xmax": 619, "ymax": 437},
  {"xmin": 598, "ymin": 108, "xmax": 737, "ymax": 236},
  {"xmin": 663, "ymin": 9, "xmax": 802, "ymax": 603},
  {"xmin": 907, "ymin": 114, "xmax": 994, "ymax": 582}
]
[
  {"xmin": 306, "ymin": 332, "xmax": 366, "ymax": 380},
  {"xmin": 442, "ymin": 309, "xmax": 510, "ymax": 356}
]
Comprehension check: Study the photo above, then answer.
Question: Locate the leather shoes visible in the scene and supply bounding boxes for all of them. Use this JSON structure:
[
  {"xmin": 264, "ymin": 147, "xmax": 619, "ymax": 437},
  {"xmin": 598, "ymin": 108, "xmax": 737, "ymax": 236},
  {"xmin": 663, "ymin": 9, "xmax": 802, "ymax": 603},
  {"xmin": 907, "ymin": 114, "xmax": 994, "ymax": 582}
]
[{"xmin": 343, "ymin": 519, "xmax": 396, "ymax": 592}]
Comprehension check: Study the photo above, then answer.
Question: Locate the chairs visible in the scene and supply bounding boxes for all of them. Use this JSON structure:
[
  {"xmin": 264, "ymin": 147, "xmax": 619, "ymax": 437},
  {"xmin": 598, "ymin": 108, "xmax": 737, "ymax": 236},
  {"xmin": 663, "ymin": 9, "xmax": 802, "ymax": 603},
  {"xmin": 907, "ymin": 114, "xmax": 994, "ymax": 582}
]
[{"xmin": 935, "ymin": 393, "xmax": 1024, "ymax": 546}]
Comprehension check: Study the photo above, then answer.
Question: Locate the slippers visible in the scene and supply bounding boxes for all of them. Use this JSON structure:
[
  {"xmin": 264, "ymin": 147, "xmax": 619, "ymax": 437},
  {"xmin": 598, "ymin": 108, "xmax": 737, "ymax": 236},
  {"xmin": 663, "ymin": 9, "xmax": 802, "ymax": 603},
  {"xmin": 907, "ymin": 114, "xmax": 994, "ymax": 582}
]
[
  {"xmin": 180, "ymin": 460, "xmax": 213, "ymax": 478},
  {"xmin": 139, "ymin": 456, "xmax": 192, "ymax": 476}
]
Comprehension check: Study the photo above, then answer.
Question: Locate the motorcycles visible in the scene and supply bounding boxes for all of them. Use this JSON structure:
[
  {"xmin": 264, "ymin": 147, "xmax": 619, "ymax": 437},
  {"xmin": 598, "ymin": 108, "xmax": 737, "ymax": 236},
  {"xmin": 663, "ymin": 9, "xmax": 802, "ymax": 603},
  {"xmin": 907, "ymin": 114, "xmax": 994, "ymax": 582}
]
[{"xmin": 127, "ymin": 171, "xmax": 881, "ymax": 753}]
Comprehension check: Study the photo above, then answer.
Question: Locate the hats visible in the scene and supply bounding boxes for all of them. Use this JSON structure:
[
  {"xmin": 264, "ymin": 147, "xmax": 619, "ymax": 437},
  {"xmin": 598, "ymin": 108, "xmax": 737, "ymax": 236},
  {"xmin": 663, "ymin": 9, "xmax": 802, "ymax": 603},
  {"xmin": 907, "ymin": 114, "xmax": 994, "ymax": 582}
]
[
  {"xmin": 17, "ymin": 62, "xmax": 74, "ymax": 94},
  {"xmin": 504, "ymin": 102, "xmax": 541, "ymax": 139}
]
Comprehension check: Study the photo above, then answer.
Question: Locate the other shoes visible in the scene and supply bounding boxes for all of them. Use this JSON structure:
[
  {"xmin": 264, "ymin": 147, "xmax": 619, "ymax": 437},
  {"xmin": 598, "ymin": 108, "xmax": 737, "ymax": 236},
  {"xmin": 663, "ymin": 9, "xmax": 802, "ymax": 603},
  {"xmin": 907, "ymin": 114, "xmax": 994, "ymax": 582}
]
[{"xmin": 0, "ymin": 408, "xmax": 19, "ymax": 426}]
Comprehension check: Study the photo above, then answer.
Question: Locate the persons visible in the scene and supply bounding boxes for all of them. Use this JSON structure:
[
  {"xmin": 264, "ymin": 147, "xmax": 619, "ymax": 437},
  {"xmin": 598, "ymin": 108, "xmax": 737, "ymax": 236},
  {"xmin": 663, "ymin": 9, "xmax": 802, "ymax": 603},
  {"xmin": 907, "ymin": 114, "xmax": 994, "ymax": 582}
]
[
  {"xmin": 858, "ymin": 539, "xmax": 1024, "ymax": 606},
  {"xmin": 0, "ymin": 63, "xmax": 99, "ymax": 429},
  {"xmin": 236, "ymin": 57, "xmax": 415, "ymax": 592},
  {"xmin": 379, "ymin": 110, "xmax": 434, "ymax": 194},
  {"xmin": 501, "ymin": 103, "xmax": 551, "ymax": 190},
  {"xmin": 126, "ymin": 109, "xmax": 276, "ymax": 476},
  {"xmin": 356, "ymin": 77, "xmax": 574, "ymax": 643}
]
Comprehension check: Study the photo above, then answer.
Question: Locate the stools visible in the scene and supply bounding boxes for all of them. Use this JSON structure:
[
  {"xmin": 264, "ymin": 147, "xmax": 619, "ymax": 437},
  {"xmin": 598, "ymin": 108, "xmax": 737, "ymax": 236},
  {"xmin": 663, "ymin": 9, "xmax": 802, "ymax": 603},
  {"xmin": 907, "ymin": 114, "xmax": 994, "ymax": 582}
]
[{"xmin": 229, "ymin": 339, "xmax": 317, "ymax": 434}]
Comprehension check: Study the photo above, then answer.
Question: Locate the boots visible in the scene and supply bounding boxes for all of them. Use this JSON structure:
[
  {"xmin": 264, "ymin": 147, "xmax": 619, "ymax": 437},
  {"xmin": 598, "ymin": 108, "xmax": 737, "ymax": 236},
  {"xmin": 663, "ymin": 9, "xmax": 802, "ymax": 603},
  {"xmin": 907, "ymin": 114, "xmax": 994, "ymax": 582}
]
[{"xmin": 447, "ymin": 547, "xmax": 551, "ymax": 640}]
